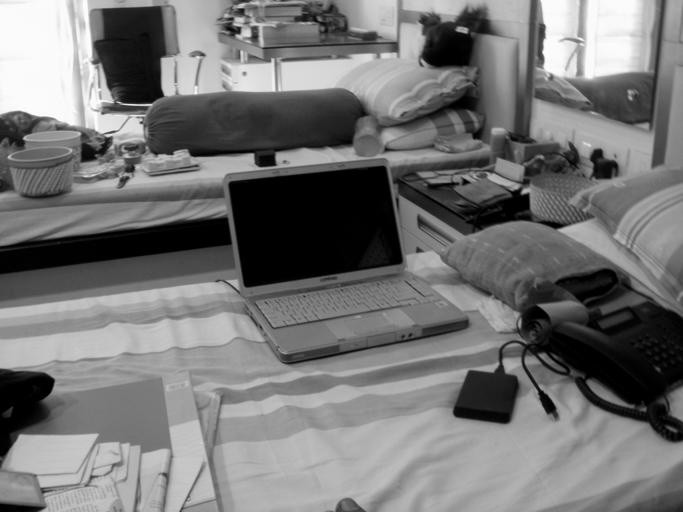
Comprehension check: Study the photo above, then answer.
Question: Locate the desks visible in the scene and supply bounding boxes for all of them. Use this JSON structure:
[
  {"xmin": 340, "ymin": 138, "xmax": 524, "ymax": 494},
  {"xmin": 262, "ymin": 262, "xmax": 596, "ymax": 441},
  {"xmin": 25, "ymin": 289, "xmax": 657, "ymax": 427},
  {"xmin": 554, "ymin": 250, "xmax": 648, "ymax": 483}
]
[{"xmin": 210, "ymin": 29, "xmax": 401, "ymax": 107}]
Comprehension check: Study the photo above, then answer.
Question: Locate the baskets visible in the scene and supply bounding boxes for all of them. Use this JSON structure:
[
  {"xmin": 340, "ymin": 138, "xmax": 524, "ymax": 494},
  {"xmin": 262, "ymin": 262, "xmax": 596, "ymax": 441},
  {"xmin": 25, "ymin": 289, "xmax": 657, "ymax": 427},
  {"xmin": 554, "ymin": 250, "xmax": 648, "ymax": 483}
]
[{"xmin": 530, "ymin": 172, "xmax": 597, "ymax": 223}]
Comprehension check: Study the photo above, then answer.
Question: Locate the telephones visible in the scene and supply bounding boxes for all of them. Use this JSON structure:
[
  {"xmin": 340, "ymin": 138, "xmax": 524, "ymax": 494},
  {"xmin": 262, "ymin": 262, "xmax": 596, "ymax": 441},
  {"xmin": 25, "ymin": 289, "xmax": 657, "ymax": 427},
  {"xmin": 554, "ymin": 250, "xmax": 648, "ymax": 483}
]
[{"xmin": 548, "ymin": 301, "xmax": 683, "ymax": 405}]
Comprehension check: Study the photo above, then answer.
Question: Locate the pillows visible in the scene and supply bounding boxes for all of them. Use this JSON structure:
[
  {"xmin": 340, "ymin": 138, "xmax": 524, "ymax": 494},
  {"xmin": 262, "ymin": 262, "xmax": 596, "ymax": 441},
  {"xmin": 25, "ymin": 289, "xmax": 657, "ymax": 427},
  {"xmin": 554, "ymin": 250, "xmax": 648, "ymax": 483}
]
[
  {"xmin": 92, "ymin": 39, "xmax": 162, "ymax": 104},
  {"xmin": 535, "ymin": 62, "xmax": 593, "ymax": 110},
  {"xmin": 379, "ymin": 104, "xmax": 485, "ymax": 151},
  {"xmin": 440, "ymin": 221, "xmax": 625, "ymax": 310},
  {"xmin": 564, "ymin": 165, "xmax": 681, "ymax": 309},
  {"xmin": 140, "ymin": 73, "xmax": 362, "ymax": 153},
  {"xmin": 335, "ymin": 52, "xmax": 478, "ymax": 126}
]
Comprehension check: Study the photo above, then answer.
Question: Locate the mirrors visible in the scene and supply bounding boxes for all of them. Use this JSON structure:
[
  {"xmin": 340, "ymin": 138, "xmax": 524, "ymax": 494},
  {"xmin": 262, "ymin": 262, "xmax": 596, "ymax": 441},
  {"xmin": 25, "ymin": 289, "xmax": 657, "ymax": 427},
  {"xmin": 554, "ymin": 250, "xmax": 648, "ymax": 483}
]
[{"xmin": 515, "ymin": 0, "xmax": 666, "ymax": 132}]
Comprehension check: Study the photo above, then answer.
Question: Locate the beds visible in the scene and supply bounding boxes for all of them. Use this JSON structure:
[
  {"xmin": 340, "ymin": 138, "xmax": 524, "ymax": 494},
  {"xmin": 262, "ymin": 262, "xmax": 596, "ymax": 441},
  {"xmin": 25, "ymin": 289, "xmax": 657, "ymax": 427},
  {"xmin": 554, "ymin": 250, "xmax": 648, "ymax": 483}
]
[
  {"xmin": 0, "ymin": 161, "xmax": 681, "ymax": 512},
  {"xmin": 0, "ymin": 19, "xmax": 518, "ymax": 275}
]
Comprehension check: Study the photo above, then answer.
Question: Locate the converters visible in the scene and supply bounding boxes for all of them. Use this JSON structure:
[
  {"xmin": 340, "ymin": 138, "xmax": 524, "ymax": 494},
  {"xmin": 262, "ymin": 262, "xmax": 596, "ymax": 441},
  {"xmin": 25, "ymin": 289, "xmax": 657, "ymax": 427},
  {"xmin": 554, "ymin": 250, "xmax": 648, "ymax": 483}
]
[{"xmin": 452, "ymin": 370, "xmax": 519, "ymax": 423}]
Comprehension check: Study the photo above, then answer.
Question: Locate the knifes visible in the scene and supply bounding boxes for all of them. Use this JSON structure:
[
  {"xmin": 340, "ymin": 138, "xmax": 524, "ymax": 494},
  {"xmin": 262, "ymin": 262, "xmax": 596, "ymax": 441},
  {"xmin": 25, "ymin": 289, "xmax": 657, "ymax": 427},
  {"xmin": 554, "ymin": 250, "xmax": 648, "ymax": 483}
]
[{"xmin": 116, "ymin": 164, "xmax": 135, "ymax": 190}]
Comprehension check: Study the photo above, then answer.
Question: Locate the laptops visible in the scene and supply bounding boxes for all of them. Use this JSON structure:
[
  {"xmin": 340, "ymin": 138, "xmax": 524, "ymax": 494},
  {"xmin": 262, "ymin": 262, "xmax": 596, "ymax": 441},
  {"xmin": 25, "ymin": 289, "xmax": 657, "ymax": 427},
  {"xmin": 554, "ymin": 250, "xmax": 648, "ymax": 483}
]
[{"xmin": 222, "ymin": 157, "xmax": 470, "ymax": 364}]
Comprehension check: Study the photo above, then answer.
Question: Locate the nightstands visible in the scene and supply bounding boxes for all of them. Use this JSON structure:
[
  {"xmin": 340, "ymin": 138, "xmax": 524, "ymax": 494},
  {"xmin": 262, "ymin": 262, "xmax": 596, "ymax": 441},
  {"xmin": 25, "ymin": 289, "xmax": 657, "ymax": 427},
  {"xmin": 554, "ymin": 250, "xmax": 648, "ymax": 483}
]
[{"xmin": 392, "ymin": 163, "xmax": 545, "ymax": 254}]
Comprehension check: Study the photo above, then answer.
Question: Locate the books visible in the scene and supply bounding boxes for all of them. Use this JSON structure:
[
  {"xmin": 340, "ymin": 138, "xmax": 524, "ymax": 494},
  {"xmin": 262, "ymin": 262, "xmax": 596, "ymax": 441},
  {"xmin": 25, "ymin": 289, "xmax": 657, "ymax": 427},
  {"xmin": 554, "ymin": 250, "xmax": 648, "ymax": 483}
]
[
  {"xmin": 218, "ymin": 1, "xmax": 303, "ymax": 45},
  {"xmin": 0, "ymin": 367, "xmax": 222, "ymax": 512}
]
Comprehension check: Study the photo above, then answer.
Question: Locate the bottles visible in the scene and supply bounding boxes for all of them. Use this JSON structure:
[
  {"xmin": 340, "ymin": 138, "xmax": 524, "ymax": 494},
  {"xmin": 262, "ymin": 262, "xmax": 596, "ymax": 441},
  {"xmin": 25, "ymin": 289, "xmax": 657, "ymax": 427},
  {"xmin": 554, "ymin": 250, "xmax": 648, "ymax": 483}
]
[{"xmin": 355, "ymin": 114, "xmax": 380, "ymax": 158}]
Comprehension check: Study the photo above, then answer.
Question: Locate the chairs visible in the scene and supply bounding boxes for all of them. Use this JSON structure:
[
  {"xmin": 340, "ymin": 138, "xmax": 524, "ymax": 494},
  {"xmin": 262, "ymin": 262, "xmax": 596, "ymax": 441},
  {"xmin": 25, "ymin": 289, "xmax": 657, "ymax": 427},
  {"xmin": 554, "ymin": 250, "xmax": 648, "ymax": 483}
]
[
  {"xmin": 546, "ymin": 34, "xmax": 583, "ymax": 72},
  {"xmin": 84, "ymin": 4, "xmax": 205, "ymax": 142}
]
